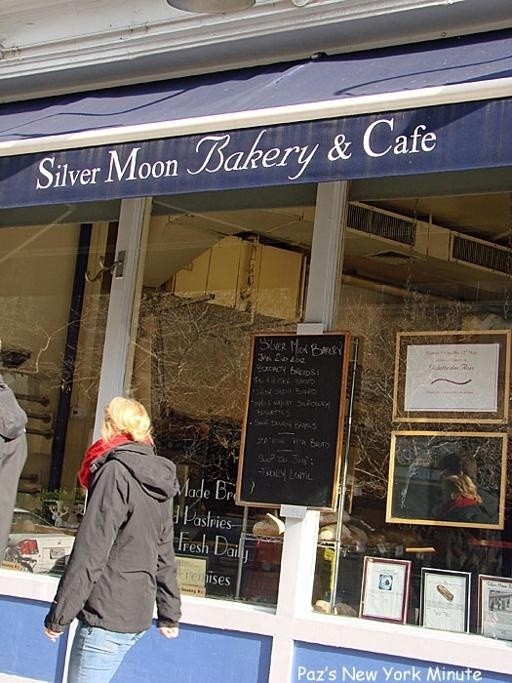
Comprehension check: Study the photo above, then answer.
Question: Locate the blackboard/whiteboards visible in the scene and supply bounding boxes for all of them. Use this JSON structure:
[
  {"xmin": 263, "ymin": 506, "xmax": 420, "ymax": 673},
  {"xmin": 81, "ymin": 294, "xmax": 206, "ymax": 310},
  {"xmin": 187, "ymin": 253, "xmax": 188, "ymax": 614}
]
[{"xmin": 235, "ymin": 333, "xmax": 351, "ymax": 514}]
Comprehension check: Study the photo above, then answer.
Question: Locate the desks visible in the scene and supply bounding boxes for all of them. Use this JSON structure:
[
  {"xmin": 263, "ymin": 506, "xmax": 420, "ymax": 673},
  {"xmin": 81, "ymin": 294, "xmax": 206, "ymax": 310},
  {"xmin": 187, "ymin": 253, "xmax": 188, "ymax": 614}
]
[
  {"xmin": 470, "ymin": 537, "xmax": 512, "ymax": 576},
  {"xmin": 246, "ymin": 528, "xmax": 402, "ymax": 604}
]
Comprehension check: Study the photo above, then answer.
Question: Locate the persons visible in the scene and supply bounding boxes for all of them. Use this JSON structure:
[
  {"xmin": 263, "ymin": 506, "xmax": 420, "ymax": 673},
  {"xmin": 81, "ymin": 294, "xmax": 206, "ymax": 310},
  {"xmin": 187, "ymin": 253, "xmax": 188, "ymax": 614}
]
[
  {"xmin": 0, "ymin": 379, "xmax": 30, "ymax": 571},
  {"xmin": 400, "ymin": 432, "xmax": 500, "ymax": 520},
  {"xmin": 420, "ymin": 470, "xmax": 505, "ymax": 634},
  {"xmin": 44, "ymin": 396, "xmax": 183, "ymax": 683}
]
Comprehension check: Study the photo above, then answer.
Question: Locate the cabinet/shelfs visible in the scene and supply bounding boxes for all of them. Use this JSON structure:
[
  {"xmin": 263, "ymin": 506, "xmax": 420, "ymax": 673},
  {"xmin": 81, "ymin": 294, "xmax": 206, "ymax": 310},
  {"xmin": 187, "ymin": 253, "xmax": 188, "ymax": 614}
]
[{"xmin": 168, "ymin": 238, "xmax": 304, "ymax": 321}]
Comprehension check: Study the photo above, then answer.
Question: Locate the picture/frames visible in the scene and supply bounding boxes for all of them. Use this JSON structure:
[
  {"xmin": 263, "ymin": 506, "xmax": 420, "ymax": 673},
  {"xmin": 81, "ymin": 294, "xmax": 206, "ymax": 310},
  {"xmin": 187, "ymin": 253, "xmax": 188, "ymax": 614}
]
[
  {"xmin": 359, "ymin": 556, "xmax": 410, "ymax": 624},
  {"xmin": 418, "ymin": 567, "xmax": 471, "ymax": 633},
  {"xmin": 174, "ymin": 554, "xmax": 209, "ymax": 598},
  {"xmin": 393, "ymin": 327, "xmax": 510, "ymax": 427},
  {"xmin": 478, "ymin": 574, "xmax": 512, "ymax": 642},
  {"xmin": 385, "ymin": 432, "xmax": 507, "ymax": 532}
]
